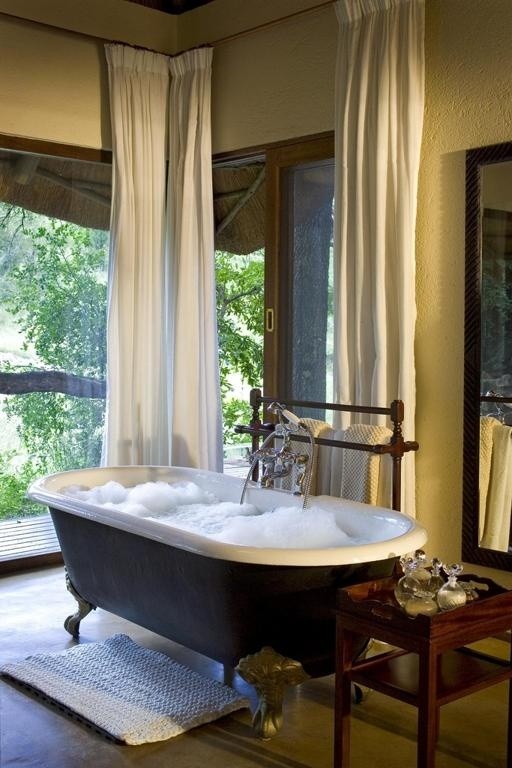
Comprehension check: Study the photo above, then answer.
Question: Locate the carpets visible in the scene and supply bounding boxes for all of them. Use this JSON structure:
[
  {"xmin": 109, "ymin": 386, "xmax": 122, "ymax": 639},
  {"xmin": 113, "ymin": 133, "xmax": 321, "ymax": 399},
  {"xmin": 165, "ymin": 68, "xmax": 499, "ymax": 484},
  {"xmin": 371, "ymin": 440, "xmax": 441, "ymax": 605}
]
[{"xmin": 1, "ymin": 634, "xmax": 251, "ymax": 747}]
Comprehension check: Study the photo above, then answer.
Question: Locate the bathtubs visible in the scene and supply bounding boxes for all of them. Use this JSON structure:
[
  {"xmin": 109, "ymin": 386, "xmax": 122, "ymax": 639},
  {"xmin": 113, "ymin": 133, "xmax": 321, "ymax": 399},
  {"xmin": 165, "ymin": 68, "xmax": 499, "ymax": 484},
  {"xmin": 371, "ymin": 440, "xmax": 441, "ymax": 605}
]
[{"xmin": 26, "ymin": 465, "xmax": 428, "ymax": 743}]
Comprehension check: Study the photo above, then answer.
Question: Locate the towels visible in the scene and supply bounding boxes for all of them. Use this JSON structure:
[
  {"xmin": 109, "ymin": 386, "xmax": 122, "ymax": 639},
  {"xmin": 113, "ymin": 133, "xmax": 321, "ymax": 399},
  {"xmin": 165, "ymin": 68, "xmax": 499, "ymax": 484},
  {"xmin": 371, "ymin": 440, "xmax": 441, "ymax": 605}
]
[
  {"xmin": 272, "ymin": 418, "xmax": 393, "ymax": 509},
  {"xmin": 479, "ymin": 416, "xmax": 512, "ymax": 552}
]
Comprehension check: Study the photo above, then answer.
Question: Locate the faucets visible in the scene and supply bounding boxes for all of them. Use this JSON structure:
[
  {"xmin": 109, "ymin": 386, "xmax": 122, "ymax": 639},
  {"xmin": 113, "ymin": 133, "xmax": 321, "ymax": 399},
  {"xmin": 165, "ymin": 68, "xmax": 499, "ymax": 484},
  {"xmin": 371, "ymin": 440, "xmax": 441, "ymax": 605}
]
[{"xmin": 256, "ymin": 463, "xmax": 292, "ymax": 487}]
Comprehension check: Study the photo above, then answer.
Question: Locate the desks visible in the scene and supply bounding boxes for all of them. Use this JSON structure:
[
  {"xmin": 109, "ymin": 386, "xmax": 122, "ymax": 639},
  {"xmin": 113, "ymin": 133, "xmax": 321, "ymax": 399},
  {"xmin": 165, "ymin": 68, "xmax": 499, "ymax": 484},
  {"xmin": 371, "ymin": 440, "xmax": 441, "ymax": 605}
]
[{"xmin": 335, "ymin": 572, "xmax": 511, "ymax": 768}]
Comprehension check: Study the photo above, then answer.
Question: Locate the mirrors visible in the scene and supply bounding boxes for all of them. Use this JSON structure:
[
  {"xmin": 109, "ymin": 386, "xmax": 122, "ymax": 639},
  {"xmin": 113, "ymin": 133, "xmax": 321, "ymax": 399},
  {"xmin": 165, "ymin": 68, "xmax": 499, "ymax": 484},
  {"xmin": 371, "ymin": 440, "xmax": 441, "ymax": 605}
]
[{"xmin": 461, "ymin": 140, "xmax": 512, "ymax": 572}]
